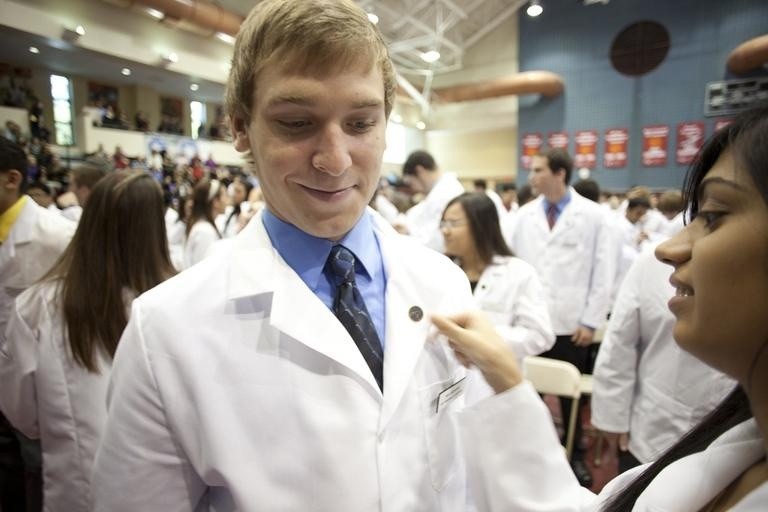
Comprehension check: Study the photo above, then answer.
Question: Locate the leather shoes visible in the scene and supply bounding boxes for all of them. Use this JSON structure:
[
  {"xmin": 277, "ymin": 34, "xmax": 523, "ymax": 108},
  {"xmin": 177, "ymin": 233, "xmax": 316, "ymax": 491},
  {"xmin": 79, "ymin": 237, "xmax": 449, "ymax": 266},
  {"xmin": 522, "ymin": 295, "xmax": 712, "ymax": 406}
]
[{"xmin": 572, "ymin": 458, "xmax": 593, "ymax": 487}]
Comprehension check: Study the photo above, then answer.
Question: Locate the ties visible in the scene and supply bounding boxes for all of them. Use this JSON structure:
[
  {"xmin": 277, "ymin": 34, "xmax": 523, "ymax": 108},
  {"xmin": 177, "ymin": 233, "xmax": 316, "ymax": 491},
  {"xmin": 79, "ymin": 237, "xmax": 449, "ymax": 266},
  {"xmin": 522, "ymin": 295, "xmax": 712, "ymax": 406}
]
[
  {"xmin": 546, "ymin": 203, "xmax": 558, "ymax": 228},
  {"xmin": 324, "ymin": 246, "xmax": 382, "ymax": 392}
]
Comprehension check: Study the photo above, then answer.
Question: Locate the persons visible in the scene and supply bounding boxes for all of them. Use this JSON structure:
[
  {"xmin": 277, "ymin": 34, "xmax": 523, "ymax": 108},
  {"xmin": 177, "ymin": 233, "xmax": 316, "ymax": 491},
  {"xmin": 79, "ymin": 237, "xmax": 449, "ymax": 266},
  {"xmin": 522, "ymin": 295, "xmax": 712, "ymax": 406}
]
[
  {"xmin": 87, "ymin": 1, "xmax": 499, "ymax": 512},
  {"xmin": 0, "ymin": 34, "xmax": 766, "ymax": 512}
]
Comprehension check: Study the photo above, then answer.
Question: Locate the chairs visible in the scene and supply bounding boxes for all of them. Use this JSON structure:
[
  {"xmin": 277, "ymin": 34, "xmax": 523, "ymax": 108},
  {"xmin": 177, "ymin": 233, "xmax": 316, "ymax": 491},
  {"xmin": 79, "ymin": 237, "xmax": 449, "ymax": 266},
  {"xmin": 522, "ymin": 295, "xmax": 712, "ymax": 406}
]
[{"xmin": 528, "ymin": 354, "xmax": 600, "ymax": 462}]
